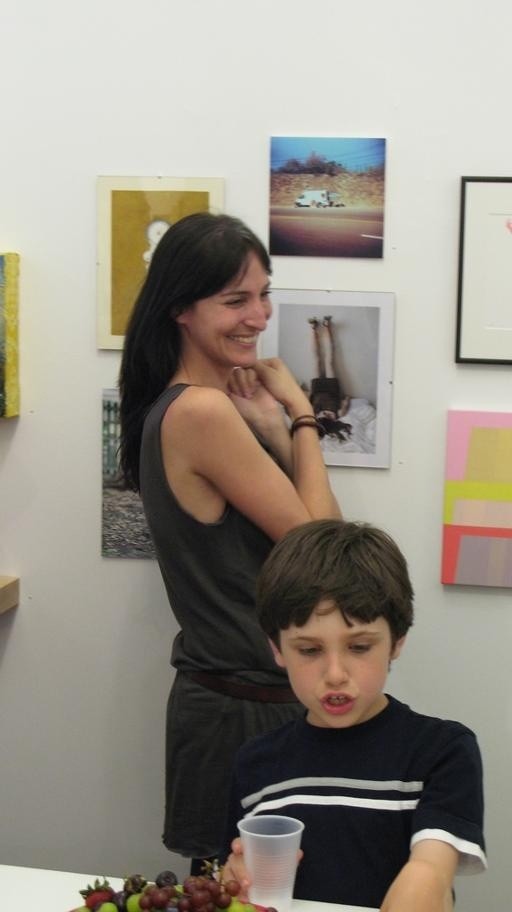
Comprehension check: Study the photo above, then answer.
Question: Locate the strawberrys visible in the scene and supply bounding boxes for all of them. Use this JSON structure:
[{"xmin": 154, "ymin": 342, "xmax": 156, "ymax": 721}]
[{"xmin": 79, "ymin": 876, "xmax": 116, "ymax": 909}]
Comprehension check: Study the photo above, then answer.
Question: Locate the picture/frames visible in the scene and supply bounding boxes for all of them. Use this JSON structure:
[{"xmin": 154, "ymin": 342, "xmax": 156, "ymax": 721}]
[
  {"xmin": 456, "ymin": 176, "xmax": 512, "ymax": 366},
  {"xmin": 261, "ymin": 289, "xmax": 392, "ymax": 470},
  {"xmin": 0, "ymin": 254, "xmax": 21, "ymax": 420},
  {"xmin": 94, "ymin": 175, "xmax": 225, "ymax": 351}
]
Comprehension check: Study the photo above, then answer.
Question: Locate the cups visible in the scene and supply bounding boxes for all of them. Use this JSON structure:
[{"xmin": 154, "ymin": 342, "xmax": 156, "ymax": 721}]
[{"xmin": 237, "ymin": 816, "xmax": 304, "ymax": 911}]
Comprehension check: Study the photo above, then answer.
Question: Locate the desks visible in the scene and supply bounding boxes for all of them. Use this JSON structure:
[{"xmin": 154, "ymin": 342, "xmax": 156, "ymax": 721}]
[{"xmin": 0, "ymin": 864, "xmax": 381, "ymax": 910}]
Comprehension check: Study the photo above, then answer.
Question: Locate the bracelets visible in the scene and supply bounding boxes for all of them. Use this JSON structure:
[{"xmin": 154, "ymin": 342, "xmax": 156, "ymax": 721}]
[
  {"xmin": 291, "ymin": 413, "xmax": 321, "ymax": 426},
  {"xmin": 293, "ymin": 422, "xmax": 325, "ymax": 442}
]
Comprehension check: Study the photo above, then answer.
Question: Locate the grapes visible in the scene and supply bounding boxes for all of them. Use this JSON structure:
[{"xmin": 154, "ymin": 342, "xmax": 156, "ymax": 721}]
[{"xmin": 139, "ymin": 861, "xmax": 240, "ymax": 911}]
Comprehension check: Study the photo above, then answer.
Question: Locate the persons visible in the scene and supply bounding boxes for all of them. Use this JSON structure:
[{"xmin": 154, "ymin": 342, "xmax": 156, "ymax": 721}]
[
  {"xmin": 115, "ymin": 211, "xmax": 344, "ymax": 876},
  {"xmin": 300, "ymin": 315, "xmax": 352, "ymax": 444},
  {"xmin": 218, "ymin": 518, "xmax": 489, "ymax": 911}
]
